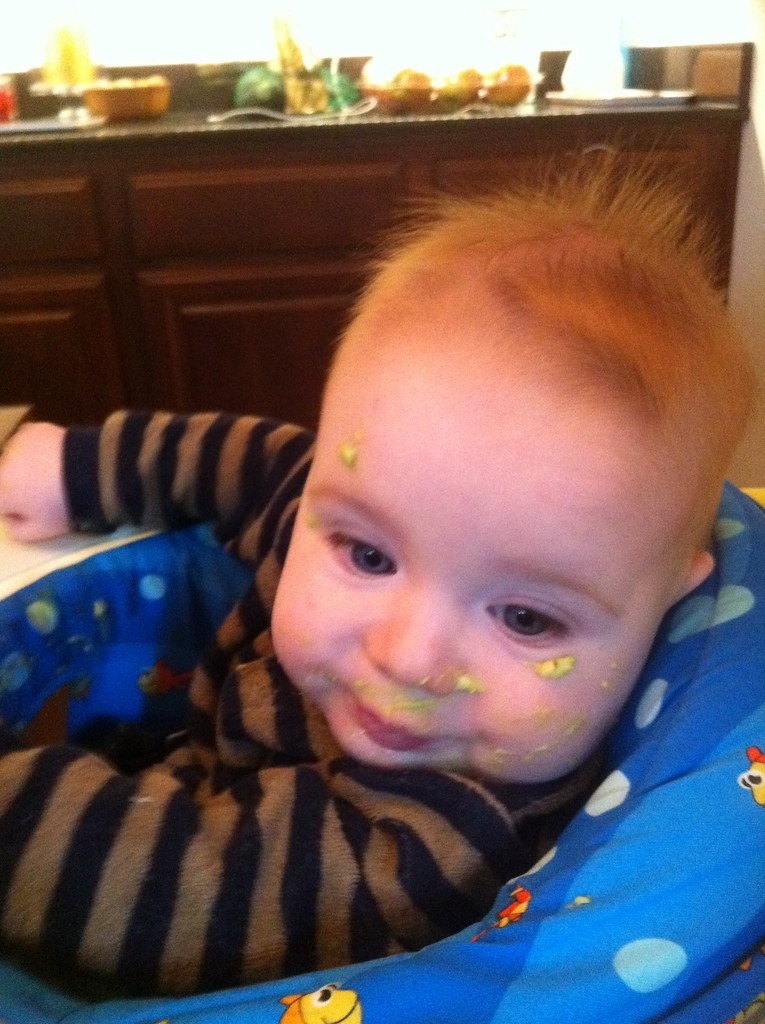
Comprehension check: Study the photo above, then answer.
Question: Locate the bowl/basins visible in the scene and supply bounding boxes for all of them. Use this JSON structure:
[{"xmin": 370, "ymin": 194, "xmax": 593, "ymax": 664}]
[{"xmin": 80, "ymin": 83, "xmax": 171, "ymax": 122}]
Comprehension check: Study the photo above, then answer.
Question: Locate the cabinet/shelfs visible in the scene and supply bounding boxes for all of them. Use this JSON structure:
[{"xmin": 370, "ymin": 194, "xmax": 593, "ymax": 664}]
[{"xmin": 0, "ymin": 38, "xmax": 752, "ymax": 437}]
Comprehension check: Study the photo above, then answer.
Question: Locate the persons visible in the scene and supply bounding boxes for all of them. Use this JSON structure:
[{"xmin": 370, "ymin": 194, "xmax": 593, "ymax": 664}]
[{"xmin": 1, "ymin": 165, "xmax": 753, "ymax": 994}]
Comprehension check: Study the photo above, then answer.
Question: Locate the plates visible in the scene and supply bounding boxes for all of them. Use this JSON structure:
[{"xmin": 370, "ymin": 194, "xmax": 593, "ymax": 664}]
[{"xmin": 351, "ymin": 74, "xmax": 545, "ymax": 116}]
